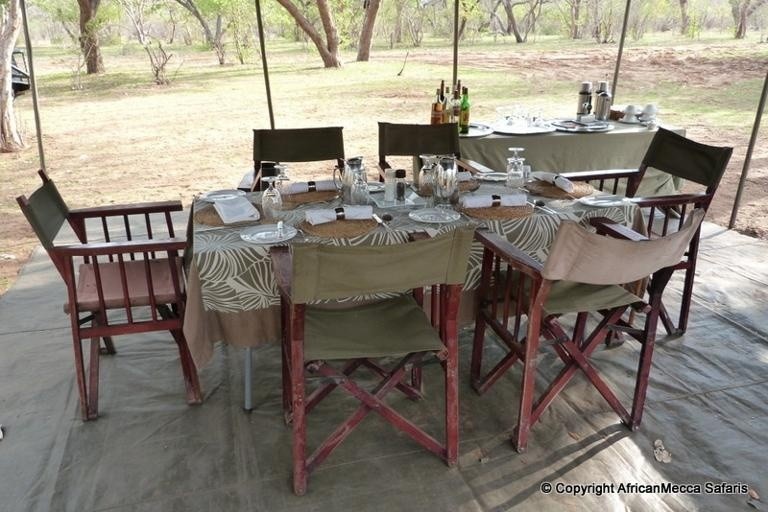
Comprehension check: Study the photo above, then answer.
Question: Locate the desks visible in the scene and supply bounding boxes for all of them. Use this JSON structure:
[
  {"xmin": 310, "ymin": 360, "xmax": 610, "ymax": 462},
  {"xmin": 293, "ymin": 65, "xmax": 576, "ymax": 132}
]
[{"xmin": 176, "ymin": 173, "xmax": 651, "ymax": 414}]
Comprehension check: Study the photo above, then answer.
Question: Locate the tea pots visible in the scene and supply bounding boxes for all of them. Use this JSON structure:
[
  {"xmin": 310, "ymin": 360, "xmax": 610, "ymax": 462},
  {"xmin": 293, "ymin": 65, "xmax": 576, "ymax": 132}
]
[
  {"xmin": 437, "ymin": 153, "xmax": 460, "ymax": 204},
  {"xmin": 595, "ymin": 80, "xmax": 612, "ymax": 120},
  {"xmin": 576, "ymin": 82, "xmax": 592, "ymax": 116}
]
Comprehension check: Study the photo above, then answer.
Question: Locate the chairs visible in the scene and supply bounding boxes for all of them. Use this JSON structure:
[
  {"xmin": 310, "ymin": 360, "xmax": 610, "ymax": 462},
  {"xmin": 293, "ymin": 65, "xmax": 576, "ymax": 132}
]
[
  {"xmin": 378, "ymin": 120, "xmax": 495, "ymax": 185},
  {"xmin": 558, "ymin": 126, "xmax": 734, "ymax": 338},
  {"xmin": 468, "ymin": 208, "xmax": 708, "ymax": 455},
  {"xmin": 268, "ymin": 225, "xmax": 473, "ymax": 495},
  {"xmin": 14, "ymin": 169, "xmax": 201, "ymax": 423},
  {"xmin": 253, "ymin": 127, "xmax": 345, "ymax": 190}
]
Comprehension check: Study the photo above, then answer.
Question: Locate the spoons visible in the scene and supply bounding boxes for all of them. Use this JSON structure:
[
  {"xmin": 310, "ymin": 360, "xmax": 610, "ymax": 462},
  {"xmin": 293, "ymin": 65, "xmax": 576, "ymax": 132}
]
[{"xmin": 533, "ymin": 198, "xmax": 561, "ymax": 213}]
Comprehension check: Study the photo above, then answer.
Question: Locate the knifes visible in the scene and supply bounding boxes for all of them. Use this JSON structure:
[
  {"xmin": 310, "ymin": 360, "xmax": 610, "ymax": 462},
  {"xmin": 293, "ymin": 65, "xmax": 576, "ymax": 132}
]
[
  {"xmin": 293, "ymin": 220, "xmax": 309, "ymax": 242},
  {"xmin": 275, "ymin": 221, "xmax": 284, "ymax": 240},
  {"xmin": 372, "ymin": 213, "xmax": 393, "ymax": 233},
  {"xmin": 525, "ymin": 199, "xmax": 553, "ymax": 215}
]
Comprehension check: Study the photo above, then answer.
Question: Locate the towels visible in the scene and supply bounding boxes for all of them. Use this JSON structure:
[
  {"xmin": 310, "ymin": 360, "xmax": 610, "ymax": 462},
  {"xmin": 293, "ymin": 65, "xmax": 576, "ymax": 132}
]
[
  {"xmin": 212, "ymin": 196, "xmax": 260, "ymax": 224},
  {"xmin": 286, "ymin": 180, "xmax": 335, "ymax": 194},
  {"xmin": 532, "ymin": 171, "xmax": 574, "ymax": 193},
  {"xmin": 463, "ymin": 193, "xmax": 527, "ymax": 209},
  {"xmin": 305, "ymin": 204, "xmax": 373, "ymax": 226}
]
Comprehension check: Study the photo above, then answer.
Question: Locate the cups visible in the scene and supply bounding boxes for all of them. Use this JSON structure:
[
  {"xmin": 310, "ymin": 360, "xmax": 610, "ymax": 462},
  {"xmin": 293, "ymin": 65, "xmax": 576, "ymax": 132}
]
[
  {"xmin": 274, "ymin": 164, "xmax": 296, "ymax": 208},
  {"xmin": 507, "ymin": 148, "xmax": 525, "ymax": 172},
  {"xmin": 261, "ymin": 176, "xmax": 282, "ymax": 218},
  {"xmin": 507, "ymin": 159, "xmax": 524, "ymax": 192},
  {"xmin": 351, "ymin": 169, "xmax": 370, "ymax": 205},
  {"xmin": 419, "ymin": 154, "xmax": 437, "ymax": 197}
]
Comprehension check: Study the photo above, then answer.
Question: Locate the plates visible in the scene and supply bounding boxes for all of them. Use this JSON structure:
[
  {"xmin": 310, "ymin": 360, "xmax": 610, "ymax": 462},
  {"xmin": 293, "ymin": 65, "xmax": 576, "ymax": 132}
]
[
  {"xmin": 545, "ymin": 118, "xmax": 614, "ymax": 132},
  {"xmin": 371, "ymin": 188, "xmax": 428, "ymax": 208},
  {"xmin": 619, "ymin": 119, "xmax": 639, "ymax": 123},
  {"xmin": 408, "ymin": 208, "xmax": 460, "ymax": 223},
  {"xmin": 454, "ymin": 122, "xmax": 495, "ymax": 137},
  {"xmin": 492, "ymin": 123, "xmax": 555, "ymax": 135},
  {"xmin": 368, "ymin": 181, "xmax": 385, "ymax": 190},
  {"xmin": 200, "ymin": 190, "xmax": 246, "ymax": 201},
  {"xmin": 474, "ymin": 172, "xmax": 506, "ymax": 181},
  {"xmin": 579, "ymin": 195, "xmax": 630, "ymax": 206},
  {"xmin": 240, "ymin": 225, "xmax": 296, "ymax": 243}
]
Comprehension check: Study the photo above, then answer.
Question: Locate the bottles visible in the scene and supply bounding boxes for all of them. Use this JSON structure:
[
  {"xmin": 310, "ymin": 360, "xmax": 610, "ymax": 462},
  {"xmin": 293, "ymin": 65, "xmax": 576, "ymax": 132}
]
[
  {"xmin": 384, "ymin": 169, "xmax": 395, "ymax": 205},
  {"xmin": 431, "ymin": 88, "xmax": 442, "ymax": 125},
  {"xmin": 450, "ymin": 85, "xmax": 457, "ymax": 100},
  {"xmin": 593, "ymin": 81, "xmax": 607, "ymax": 114},
  {"xmin": 440, "ymin": 80, "xmax": 447, "ymax": 124},
  {"xmin": 395, "ymin": 169, "xmax": 406, "ymax": 205},
  {"xmin": 595, "ymin": 92, "xmax": 610, "ymax": 121},
  {"xmin": 457, "ymin": 79, "xmax": 462, "ymax": 103},
  {"xmin": 577, "ymin": 82, "xmax": 591, "ymax": 121},
  {"xmin": 459, "ymin": 88, "xmax": 470, "ymax": 134},
  {"xmin": 451, "ymin": 90, "xmax": 461, "ymax": 131},
  {"xmin": 445, "ymin": 87, "xmax": 450, "ymax": 122}
]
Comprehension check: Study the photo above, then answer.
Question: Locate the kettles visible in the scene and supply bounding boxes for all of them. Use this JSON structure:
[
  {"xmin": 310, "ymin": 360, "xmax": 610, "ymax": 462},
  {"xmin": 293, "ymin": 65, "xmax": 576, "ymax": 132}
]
[{"xmin": 333, "ymin": 156, "xmax": 363, "ymax": 201}]
